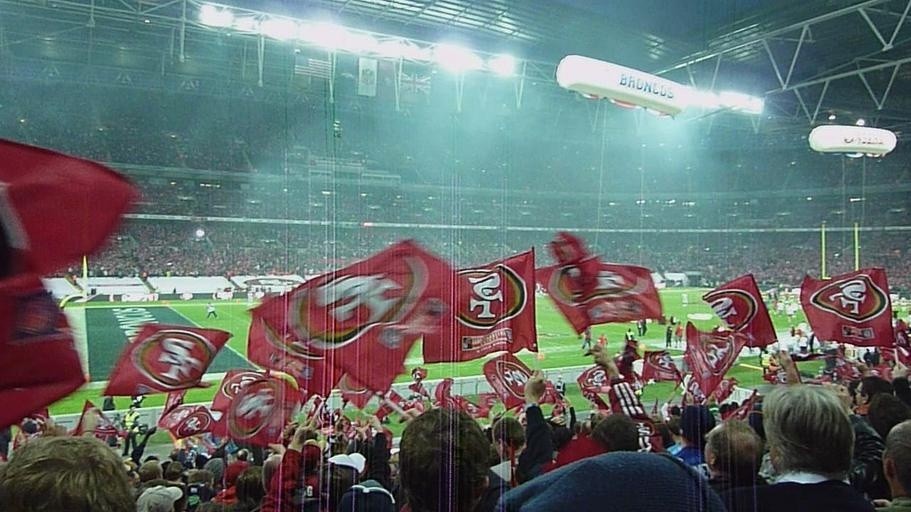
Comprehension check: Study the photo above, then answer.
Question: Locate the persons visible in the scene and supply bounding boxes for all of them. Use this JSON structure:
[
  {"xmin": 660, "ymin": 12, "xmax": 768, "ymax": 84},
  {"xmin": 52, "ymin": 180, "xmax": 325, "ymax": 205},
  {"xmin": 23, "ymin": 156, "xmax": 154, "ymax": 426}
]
[{"xmin": 1, "ymin": 119, "xmax": 911, "ymax": 512}]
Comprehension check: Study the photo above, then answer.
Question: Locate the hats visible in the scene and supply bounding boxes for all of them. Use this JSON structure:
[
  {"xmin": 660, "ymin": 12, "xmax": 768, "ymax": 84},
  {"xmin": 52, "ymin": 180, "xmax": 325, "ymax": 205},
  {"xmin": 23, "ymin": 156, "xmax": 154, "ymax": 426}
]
[
  {"xmin": 328, "ymin": 452, "xmax": 367, "ymax": 488},
  {"xmin": 135, "ymin": 485, "xmax": 184, "ymax": 512},
  {"xmin": 681, "ymin": 405, "xmax": 715, "ymax": 439}
]
